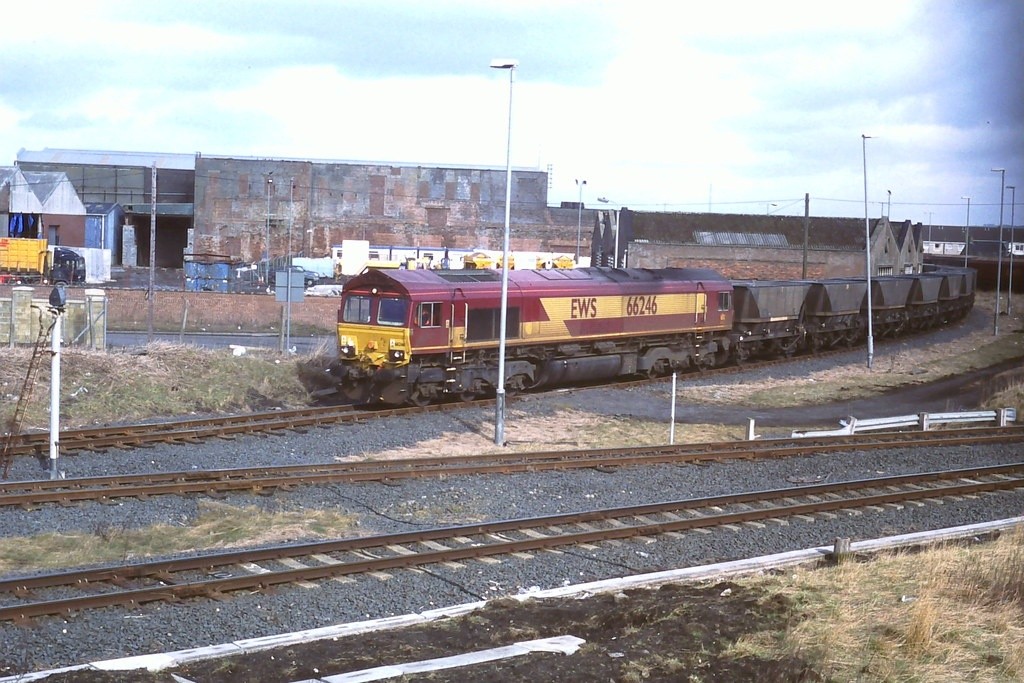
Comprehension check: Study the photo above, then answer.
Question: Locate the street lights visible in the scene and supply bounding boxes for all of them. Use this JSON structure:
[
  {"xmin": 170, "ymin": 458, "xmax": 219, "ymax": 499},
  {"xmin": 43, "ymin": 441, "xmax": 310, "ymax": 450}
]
[
  {"xmin": 925, "ymin": 210, "xmax": 936, "ymax": 254},
  {"xmin": 861, "ymin": 134, "xmax": 879, "ymax": 366},
  {"xmin": 597, "ymin": 199, "xmax": 620, "ymax": 269},
  {"xmin": 1004, "ymin": 186, "xmax": 1016, "ymax": 314},
  {"xmin": 286, "ymin": 177, "xmax": 296, "ymax": 355},
  {"xmin": 960, "ymin": 194, "xmax": 970, "ymax": 267},
  {"xmin": 266, "ymin": 178, "xmax": 272, "ymax": 284},
  {"xmin": 766, "ymin": 203, "xmax": 777, "ymax": 233},
  {"xmin": 991, "ymin": 168, "xmax": 1006, "ymax": 334},
  {"xmin": 575, "ymin": 178, "xmax": 588, "ymax": 264},
  {"xmin": 490, "ymin": 58, "xmax": 518, "ymax": 444}
]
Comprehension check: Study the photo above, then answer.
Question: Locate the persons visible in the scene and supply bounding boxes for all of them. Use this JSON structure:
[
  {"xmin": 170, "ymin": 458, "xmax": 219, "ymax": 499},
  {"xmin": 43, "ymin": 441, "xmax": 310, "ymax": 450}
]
[{"xmin": 421, "ymin": 307, "xmax": 431, "ymax": 326}]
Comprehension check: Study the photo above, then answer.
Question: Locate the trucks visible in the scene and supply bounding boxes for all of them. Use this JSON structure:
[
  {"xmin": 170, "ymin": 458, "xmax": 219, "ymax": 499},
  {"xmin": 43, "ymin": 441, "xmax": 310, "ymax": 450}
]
[{"xmin": 0, "ymin": 237, "xmax": 87, "ymax": 290}]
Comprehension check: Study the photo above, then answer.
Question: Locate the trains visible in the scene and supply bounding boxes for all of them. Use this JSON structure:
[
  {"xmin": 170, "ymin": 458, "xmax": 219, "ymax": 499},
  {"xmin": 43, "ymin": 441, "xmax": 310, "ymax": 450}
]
[{"xmin": 335, "ymin": 262, "xmax": 977, "ymax": 398}]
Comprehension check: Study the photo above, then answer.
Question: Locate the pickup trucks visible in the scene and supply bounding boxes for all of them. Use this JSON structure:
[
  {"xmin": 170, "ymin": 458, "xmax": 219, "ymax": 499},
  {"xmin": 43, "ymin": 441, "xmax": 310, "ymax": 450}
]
[{"xmin": 267, "ymin": 264, "xmax": 319, "ymax": 286}]
